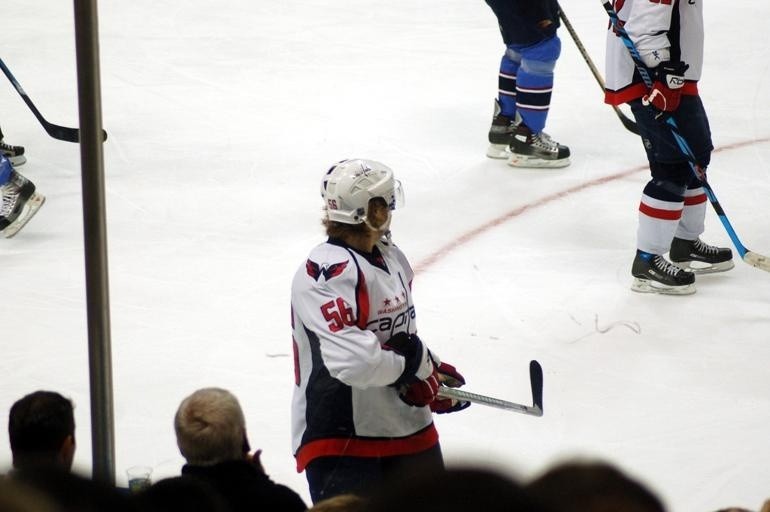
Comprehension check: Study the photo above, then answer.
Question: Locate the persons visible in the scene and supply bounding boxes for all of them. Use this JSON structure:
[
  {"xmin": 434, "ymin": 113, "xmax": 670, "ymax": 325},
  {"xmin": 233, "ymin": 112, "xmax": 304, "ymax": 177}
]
[
  {"xmin": 483, "ymin": 0, "xmax": 573, "ymax": 170},
  {"xmin": 0, "ymin": 389, "xmax": 134, "ymax": 512},
  {"xmin": 0, "ymin": 118, "xmax": 29, "ymax": 168},
  {"xmin": 128, "ymin": 386, "xmax": 311, "ymax": 512},
  {"xmin": 289, "ymin": 156, "xmax": 472, "ymax": 507},
  {"xmin": 0, "ymin": 154, "xmax": 48, "ymax": 241},
  {"xmin": 604, "ymin": 0, "xmax": 736, "ymax": 297}
]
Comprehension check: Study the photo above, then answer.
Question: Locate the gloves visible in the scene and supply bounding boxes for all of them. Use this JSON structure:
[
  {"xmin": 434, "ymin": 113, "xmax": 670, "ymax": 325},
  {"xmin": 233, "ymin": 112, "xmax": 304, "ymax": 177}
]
[
  {"xmin": 394, "ymin": 335, "xmax": 444, "ymax": 406},
  {"xmin": 643, "ymin": 71, "xmax": 684, "ymax": 112},
  {"xmin": 432, "ymin": 368, "xmax": 468, "ymax": 413}
]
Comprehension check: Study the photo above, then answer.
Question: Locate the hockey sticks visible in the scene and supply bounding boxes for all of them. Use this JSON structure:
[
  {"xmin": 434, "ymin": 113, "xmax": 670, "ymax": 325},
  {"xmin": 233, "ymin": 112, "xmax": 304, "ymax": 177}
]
[
  {"xmin": 555, "ymin": 0, "xmax": 641, "ymax": 135},
  {"xmin": 434, "ymin": 361, "xmax": 544, "ymax": 420},
  {"xmin": 603, "ymin": 0, "xmax": 770, "ymax": 271},
  {"xmin": 0, "ymin": 60, "xmax": 107, "ymax": 145}
]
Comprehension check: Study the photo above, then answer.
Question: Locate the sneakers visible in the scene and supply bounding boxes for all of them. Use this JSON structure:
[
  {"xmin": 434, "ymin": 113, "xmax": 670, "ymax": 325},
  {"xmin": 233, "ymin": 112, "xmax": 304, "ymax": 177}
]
[
  {"xmin": 489, "ymin": 110, "xmax": 516, "ymax": 147},
  {"xmin": 670, "ymin": 238, "xmax": 735, "ymax": 262},
  {"xmin": 0, "ymin": 136, "xmax": 25, "ymax": 153},
  {"xmin": 513, "ymin": 126, "xmax": 572, "ymax": 155},
  {"xmin": 4, "ymin": 177, "xmax": 34, "ymax": 230},
  {"xmin": 630, "ymin": 255, "xmax": 695, "ymax": 283}
]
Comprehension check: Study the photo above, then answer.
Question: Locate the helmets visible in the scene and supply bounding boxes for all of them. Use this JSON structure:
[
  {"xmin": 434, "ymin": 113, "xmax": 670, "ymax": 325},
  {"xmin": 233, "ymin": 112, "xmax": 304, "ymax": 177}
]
[{"xmin": 322, "ymin": 158, "xmax": 405, "ymax": 236}]
